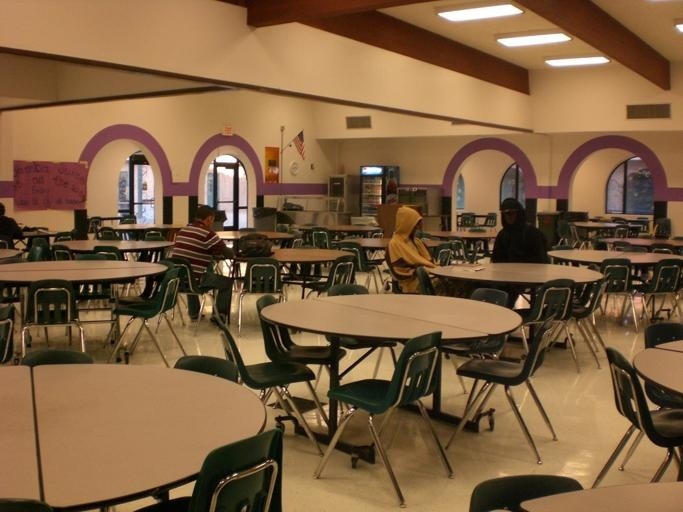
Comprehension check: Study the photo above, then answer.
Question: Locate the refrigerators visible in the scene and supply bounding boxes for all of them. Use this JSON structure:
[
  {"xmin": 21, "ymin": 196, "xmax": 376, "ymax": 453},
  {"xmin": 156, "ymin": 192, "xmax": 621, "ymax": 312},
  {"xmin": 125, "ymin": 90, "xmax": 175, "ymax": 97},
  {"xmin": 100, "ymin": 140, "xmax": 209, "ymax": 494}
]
[{"xmin": 359, "ymin": 166, "xmax": 400, "ymax": 217}]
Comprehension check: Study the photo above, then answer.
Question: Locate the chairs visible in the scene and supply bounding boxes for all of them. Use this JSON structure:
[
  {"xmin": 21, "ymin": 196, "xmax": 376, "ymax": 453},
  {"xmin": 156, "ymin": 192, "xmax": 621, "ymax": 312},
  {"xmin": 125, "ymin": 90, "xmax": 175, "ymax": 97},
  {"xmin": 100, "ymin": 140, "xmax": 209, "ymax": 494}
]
[
  {"xmin": 648, "ymin": 242, "xmax": 678, "ymax": 254},
  {"xmin": 636, "ymin": 217, "xmax": 649, "ymax": 233},
  {"xmin": 385, "ymin": 246, "xmax": 450, "ymax": 295},
  {"xmin": 622, "ymin": 246, "xmax": 648, "ymax": 252},
  {"xmin": 316, "ymin": 283, "xmax": 396, "ymax": 379},
  {"xmin": 273, "ymin": 224, "xmax": 288, "ymax": 249},
  {"xmin": 95, "ymin": 227, "xmax": 117, "ymax": 240},
  {"xmin": 640, "ymin": 259, "xmax": 682, "ymax": 323},
  {"xmin": 513, "ymin": 278, "xmax": 580, "ymax": 373},
  {"xmin": 615, "ymin": 225, "xmax": 628, "ymax": 238},
  {"xmin": 209, "ymin": 315, "xmax": 329, "ymax": 457},
  {"xmin": 120, "ymin": 215, "xmax": 136, "ymax": 224},
  {"xmin": 456, "ymin": 215, "xmax": 465, "ymax": 232},
  {"xmin": 26, "ymin": 245, "xmax": 51, "ymax": 262},
  {"xmin": 0, "ymin": 498, "xmax": 54, "ymax": 512},
  {"xmin": 137, "ymin": 228, "xmax": 165, "ymax": 262},
  {"xmin": 70, "ymin": 253, "xmax": 115, "ymax": 348},
  {"xmin": 102, "ymin": 261, "xmax": 174, "ymax": 348},
  {"xmin": 478, "ymin": 213, "xmax": 496, "ymax": 229},
  {"xmin": 21, "ymin": 279, "xmax": 85, "ymax": 357},
  {"xmin": 31, "ymin": 237, "xmax": 48, "ymax": 248},
  {"xmin": 599, "ymin": 258, "xmax": 638, "ymax": 333},
  {"xmin": 51, "ymin": 244, "xmax": 74, "ymax": 261},
  {"xmin": 590, "ymin": 346, "xmax": 683, "ymax": 488},
  {"xmin": 448, "ymin": 240, "xmax": 465, "ymax": 265},
  {"xmin": 460, "ymin": 212, "xmax": 475, "ymax": 231},
  {"xmin": 613, "ymin": 241, "xmax": 631, "ymax": 250},
  {"xmin": 572, "ymin": 263, "xmax": 599, "ymax": 352},
  {"xmin": 673, "ymin": 236, "xmax": 683, "ymax": 240},
  {"xmin": 133, "ymin": 428, "xmax": 282, "ymax": 512},
  {"xmin": 88, "ymin": 216, "xmax": 101, "ymax": 233},
  {"xmin": 469, "ymin": 474, "xmax": 583, "ymax": 512},
  {"xmin": 644, "ymin": 322, "xmax": 683, "ymax": 465},
  {"xmin": 53, "ymin": 232, "xmax": 72, "ymax": 242},
  {"xmin": 308, "ymin": 227, "xmax": 331, "ymax": 248},
  {"xmin": 19, "ymin": 348, "xmax": 93, "ymax": 366},
  {"xmin": 21, "ymin": 227, "xmax": 49, "ymax": 251},
  {"xmin": 238, "ymin": 257, "xmax": 287, "ymax": 332},
  {"xmin": 286, "ymin": 227, "xmax": 302, "ymax": 249},
  {"xmin": 301, "ymin": 255, "xmax": 357, "ymax": 299},
  {"xmin": 438, "ymin": 287, "xmax": 508, "ymax": 394},
  {"xmin": 331, "ymin": 242, "xmax": 383, "ymax": 294},
  {"xmin": 166, "ymin": 257, "xmax": 226, "ymax": 327},
  {"xmin": 0, "ymin": 304, "xmax": 14, "ymax": 363},
  {"xmin": 92, "ymin": 245, "xmax": 124, "ymax": 259},
  {"xmin": 351, "ymin": 223, "xmax": 368, "ymax": 238},
  {"xmin": 611, "ymin": 216, "xmax": 627, "ymax": 224},
  {"xmin": 108, "ymin": 267, "xmax": 187, "ymax": 368},
  {"xmin": 653, "ymin": 232, "xmax": 669, "ymax": 240},
  {"xmin": 638, "ymin": 218, "xmax": 670, "ymax": 237},
  {"xmin": 550, "ymin": 280, "xmax": 606, "ymax": 369},
  {"xmin": 255, "ymin": 294, "xmax": 346, "ymax": 414},
  {"xmin": 569, "ymin": 224, "xmax": 592, "ymax": 249}
]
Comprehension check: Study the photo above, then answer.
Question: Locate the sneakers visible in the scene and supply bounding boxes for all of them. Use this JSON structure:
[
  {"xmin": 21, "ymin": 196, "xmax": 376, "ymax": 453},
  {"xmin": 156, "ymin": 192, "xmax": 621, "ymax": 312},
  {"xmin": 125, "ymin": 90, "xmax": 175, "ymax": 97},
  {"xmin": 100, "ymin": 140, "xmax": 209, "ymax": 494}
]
[
  {"xmin": 210, "ymin": 314, "xmax": 226, "ymax": 322},
  {"xmin": 192, "ymin": 313, "xmax": 205, "ymax": 321}
]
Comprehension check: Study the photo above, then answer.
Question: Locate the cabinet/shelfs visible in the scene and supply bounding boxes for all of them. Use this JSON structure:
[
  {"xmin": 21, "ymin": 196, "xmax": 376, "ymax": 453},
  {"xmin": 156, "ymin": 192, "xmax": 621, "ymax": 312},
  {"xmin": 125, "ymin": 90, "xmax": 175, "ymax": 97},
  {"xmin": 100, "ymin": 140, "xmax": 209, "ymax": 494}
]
[
  {"xmin": 397, "ymin": 185, "xmax": 443, "ymax": 217},
  {"xmin": 538, "ymin": 210, "xmax": 588, "ymax": 252}
]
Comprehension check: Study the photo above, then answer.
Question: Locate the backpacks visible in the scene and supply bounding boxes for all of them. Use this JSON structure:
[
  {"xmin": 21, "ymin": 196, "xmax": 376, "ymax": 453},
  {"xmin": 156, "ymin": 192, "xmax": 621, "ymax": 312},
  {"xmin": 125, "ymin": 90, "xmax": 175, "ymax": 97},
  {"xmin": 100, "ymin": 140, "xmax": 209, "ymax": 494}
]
[{"xmin": 232, "ymin": 233, "xmax": 276, "ymax": 258}]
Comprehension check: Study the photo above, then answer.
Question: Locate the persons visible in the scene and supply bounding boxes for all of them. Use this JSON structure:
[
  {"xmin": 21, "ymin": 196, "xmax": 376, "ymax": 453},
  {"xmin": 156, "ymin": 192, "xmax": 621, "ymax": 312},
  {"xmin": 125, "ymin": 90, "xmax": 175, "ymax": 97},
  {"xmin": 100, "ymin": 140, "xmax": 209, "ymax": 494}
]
[
  {"xmin": 489, "ymin": 197, "xmax": 552, "ymax": 310},
  {"xmin": 388, "ymin": 206, "xmax": 446, "ymax": 295},
  {"xmin": 170, "ymin": 205, "xmax": 232, "ymax": 324},
  {"xmin": 1, "ymin": 204, "xmax": 25, "ymax": 248}
]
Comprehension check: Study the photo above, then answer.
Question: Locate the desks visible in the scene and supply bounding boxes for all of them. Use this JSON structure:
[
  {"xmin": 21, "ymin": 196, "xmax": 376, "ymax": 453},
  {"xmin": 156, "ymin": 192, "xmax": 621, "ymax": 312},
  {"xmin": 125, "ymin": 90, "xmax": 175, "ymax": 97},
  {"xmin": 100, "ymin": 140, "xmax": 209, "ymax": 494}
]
[
  {"xmin": 51, "ymin": 240, "xmax": 175, "ymax": 263},
  {"xmin": 96, "ymin": 216, "xmax": 122, "ymax": 220},
  {"xmin": 259, "ymin": 293, "xmax": 522, "ymax": 468},
  {"xmin": 632, "ymin": 340, "xmax": 683, "ymax": 394},
  {"xmin": 336, "ymin": 238, "xmax": 453, "ymax": 249},
  {"xmin": 546, "ymin": 249, "xmax": 682, "ymax": 263},
  {"xmin": 231, "ymin": 247, "xmax": 355, "ymax": 299},
  {"xmin": 0, "ymin": 363, "xmax": 266, "ymax": 511},
  {"xmin": 569, "ymin": 221, "xmax": 642, "ymax": 229},
  {"xmin": 466, "ymin": 214, "xmax": 488, "ymax": 217},
  {"xmin": 214, "ymin": 230, "xmax": 295, "ymax": 248},
  {"xmin": 597, "ymin": 237, "xmax": 683, "ymax": 247},
  {"xmin": 0, "ymin": 248, "xmax": 19, "ymax": 259},
  {"xmin": 520, "ymin": 481, "xmax": 683, "ymax": 511},
  {"xmin": 106, "ymin": 224, "xmax": 186, "ymax": 240},
  {"xmin": 609, "ymin": 219, "xmax": 650, "ymax": 232},
  {"xmin": 295, "ymin": 225, "xmax": 381, "ymax": 238},
  {"xmin": 22, "ymin": 230, "xmax": 57, "ymax": 238},
  {"xmin": 425, "ymin": 261, "xmax": 604, "ymax": 349},
  {"xmin": 422, "ymin": 230, "xmax": 496, "ymax": 257},
  {"xmin": 0, "ymin": 260, "xmax": 168, "ymax": 363}
]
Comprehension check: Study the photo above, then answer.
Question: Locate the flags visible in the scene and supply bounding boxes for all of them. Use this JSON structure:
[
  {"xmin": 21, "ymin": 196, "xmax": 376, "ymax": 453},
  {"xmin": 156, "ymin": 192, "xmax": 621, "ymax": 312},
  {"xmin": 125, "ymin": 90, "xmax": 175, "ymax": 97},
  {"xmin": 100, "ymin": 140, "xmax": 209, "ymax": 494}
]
[{"xmin": 293, "ymin": 130, "xmax": 306, "ymax": 160}]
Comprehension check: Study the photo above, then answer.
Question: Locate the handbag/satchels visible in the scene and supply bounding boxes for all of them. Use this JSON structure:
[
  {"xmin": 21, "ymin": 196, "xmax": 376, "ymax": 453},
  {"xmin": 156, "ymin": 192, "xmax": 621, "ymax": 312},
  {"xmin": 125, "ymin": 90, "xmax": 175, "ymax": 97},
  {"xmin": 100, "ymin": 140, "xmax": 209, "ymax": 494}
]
[{"xmin": 234, "ymin": 234, "xmax": 272, "ymax": 256}]
